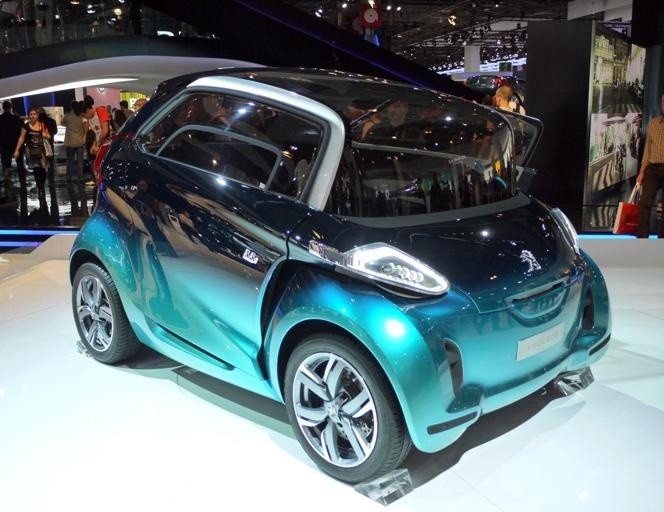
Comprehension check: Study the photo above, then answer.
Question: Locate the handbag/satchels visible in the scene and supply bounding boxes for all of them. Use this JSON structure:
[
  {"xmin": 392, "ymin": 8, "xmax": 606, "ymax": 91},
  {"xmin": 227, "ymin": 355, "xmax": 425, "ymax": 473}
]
[{"xmin": 43, "ymin": 138, "xmax": 54, "ymax": 157}]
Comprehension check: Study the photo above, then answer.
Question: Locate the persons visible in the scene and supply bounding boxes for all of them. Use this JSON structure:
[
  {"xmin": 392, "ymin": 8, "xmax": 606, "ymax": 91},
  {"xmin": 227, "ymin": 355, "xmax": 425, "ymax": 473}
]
[
  {"xmin": 361, "ymin": 85, "xmax": 527, "ymax": 169},
  {"xmin": 633, "ymin": 95, "xmax": 664, "ymax": 238},
  {"xmin": 0, "ymin": 95, "xmax": 148, "ymax": 215},
  {"xmin": 0, "ymin": 1, "xmax": 129, "ymax": 53},
  {"xmin": 186, "ymin": 99, "xmax": 265, "ymax": 144}
]
